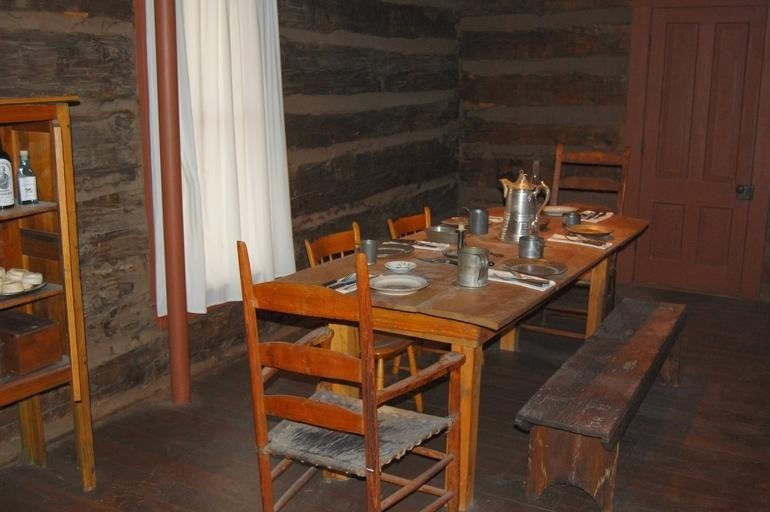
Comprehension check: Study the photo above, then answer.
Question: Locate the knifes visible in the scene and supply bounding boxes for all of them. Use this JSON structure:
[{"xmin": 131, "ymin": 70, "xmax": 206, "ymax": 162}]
[{"xmin": 330, "ymin": 273, "xmax": 380, "ymax": 291}]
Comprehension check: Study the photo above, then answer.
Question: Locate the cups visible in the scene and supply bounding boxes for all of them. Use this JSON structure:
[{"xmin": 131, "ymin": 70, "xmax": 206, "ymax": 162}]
[
  {"xmin": 469, "ymin": 208, "xmax": 489, "ymax": 235},
  {"xmin": 561, "ymin": 210, "xmax": 581, "ymax": 229},
  {"xmin": 456, "ymin": 245, "xmax": 491, "ymax": 288},
  {"xmin": 361, "ymin": 238, "xmax": 379, "ymax": 267},
  {"xmin": 518, "ymin": 236, "xmax": 544, "ymax": 259}
]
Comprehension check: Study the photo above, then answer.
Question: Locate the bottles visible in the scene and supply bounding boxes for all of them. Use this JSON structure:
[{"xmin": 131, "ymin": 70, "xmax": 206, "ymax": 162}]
[
  {"xmin": 0, "ymin": 145, "xmax": 16, "ymax": 211},
  {"xmin": 16, "ymin": 149, "xmax": 39, "ymax": 206}
]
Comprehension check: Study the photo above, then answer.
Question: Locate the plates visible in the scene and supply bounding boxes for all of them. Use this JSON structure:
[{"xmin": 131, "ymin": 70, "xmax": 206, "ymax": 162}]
[
  {"xmin": 384, "ymin": 261, "xmax": 420, "ymax": 273},
  {"xmin": 541, "ymin": 205, "xmax": 576, "ymax": 217},
  {"xmin": 369, "ymin": 273, "xmax": 432, "ymax": 294},
  {"xmin": 501, "ymin": 257, "xmax": 569, "ymax": 277},
  {"xmin": 565, "ymin": 222, "xmax": 615, "ymax": 238},
  {"xmin": 442, "ymin": 216, "xmax": 470, "ymax": 225},
  {"xmin": 377, "ymin": 243, "xmax": 416, "ymax": 259},
  {"xmin": 0, "ymin": 280, "xmax": 48, "ymax": 298},
  {"xmin": 442, "ymin": 248, "xmax": 458, "ymax": 259}
]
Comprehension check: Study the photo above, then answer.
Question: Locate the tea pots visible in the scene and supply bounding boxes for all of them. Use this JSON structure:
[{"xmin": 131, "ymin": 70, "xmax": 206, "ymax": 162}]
[{"xmin": 496, "ymin": 169, "xmax": 553, "ymax": 245}]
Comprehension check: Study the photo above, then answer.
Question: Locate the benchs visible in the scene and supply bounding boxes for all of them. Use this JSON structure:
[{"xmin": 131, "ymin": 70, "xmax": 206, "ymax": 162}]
[{"xmin": 515, "ymin": 296, "xmax": 686, "ymax": 512}]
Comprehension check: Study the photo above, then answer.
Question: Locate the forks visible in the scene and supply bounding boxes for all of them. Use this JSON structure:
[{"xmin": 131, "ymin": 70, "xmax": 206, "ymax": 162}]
[{"xmin": 320, "ymin": 274, "xmax": 351, "ymax": 289}]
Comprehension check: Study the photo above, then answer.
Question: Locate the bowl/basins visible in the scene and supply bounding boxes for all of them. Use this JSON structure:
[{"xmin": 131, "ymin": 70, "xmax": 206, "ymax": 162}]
[
  {"xmin": 538, "ymin": 216, "xmax": 551, "ymax": 232},
  {"xmin": 424, "ymin": 225, "xmax": 460, "ymax": 243}
]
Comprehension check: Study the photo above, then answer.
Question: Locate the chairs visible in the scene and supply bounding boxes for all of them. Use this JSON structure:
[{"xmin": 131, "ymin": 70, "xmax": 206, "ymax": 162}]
[
  {"xmin": 227, "ymin": 240, "xmax": 463, "ymax": 512},
  {"xmin": 304, "ymin": 220, "xmax": 429, "ymax": 413},
  {"xmin": 386, "ymin": 206, "xmax": 487, "ymax": 378},
  {"xmin": 516, "ymin": 142, "xmax": 634, "ymax": 343}
]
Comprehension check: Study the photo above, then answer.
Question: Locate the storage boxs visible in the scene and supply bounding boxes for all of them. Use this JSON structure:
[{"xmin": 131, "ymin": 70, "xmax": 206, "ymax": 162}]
[{"xmin": 1, "ymin": 310, "xmax": 63, "ymax": 376}]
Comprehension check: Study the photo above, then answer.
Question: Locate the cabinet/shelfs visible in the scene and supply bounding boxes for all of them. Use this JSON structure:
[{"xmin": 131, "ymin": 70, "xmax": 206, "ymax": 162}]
[{"xmin": 1, "ymin": 94, "xmax": 97, "ymax": 492}]
